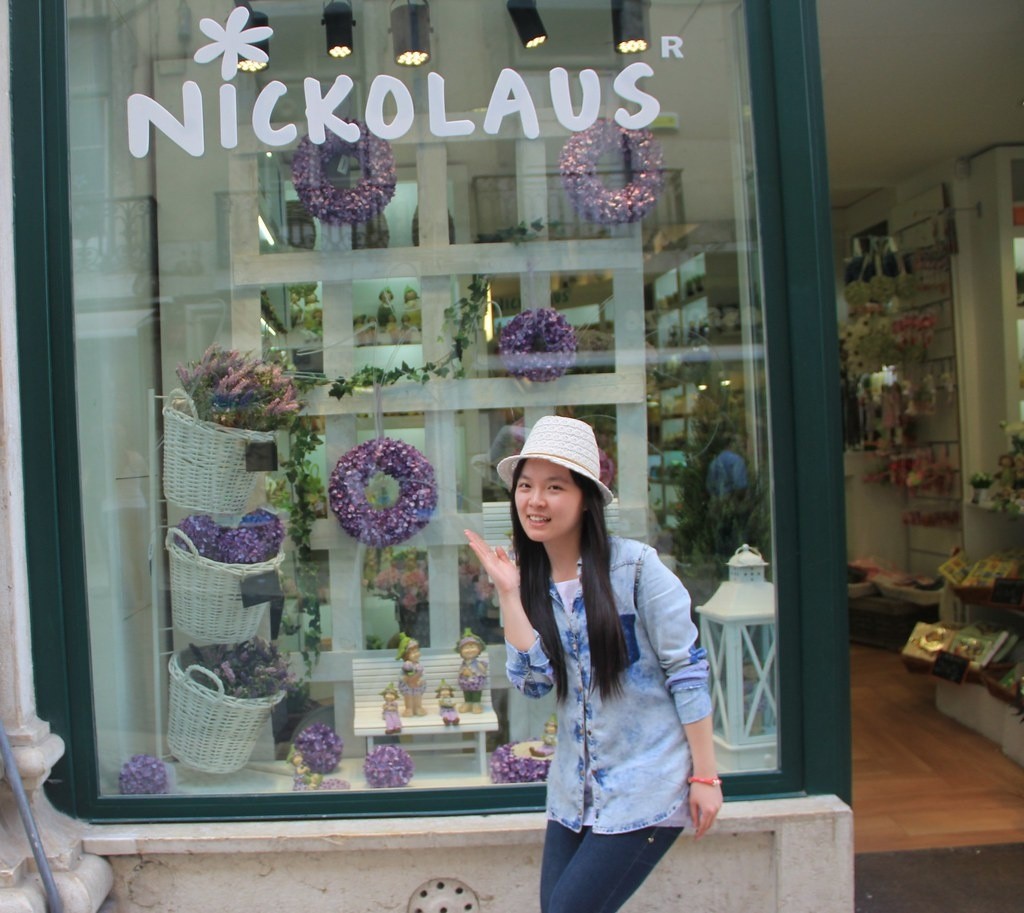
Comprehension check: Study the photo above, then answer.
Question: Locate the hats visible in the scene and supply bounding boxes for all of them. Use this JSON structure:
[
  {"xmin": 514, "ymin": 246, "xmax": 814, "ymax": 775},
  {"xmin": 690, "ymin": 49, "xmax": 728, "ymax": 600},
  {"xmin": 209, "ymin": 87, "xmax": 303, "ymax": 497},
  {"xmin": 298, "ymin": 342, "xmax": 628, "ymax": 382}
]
[
  {"xmin": 496, "ymin": 416, "xmax": 613, "ymax": 507},
  {"xmin": 470, "ymin": 425, "xmax": 525, "ymax": 488}
]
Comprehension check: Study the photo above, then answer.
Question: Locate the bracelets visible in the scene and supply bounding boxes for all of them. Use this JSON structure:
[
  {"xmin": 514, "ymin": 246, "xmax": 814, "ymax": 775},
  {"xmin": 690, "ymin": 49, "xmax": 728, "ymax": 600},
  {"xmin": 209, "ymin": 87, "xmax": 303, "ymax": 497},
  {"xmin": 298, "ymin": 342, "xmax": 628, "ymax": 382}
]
[{"xmin": 686, "ymin": 780, "xmax": 723, "ymax": 786}]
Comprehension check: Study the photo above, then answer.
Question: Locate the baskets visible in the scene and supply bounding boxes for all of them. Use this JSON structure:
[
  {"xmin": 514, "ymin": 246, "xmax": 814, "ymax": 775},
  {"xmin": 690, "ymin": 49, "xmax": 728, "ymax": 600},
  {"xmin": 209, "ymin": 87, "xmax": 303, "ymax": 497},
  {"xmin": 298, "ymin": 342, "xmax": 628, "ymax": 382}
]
[
  {"xmin": 166, "ymin": 526, "xmax": 284, "ymax": 644},
  {"xmin": 848, "ymin": 582, "xmax": 877, "ymax": 598},
  {"xmin": 879, "ymin": 582, "xmax": 940, "ymax": 605},
  {"xmin": 161, "ymin": 388, "xmax": 277, "ymax": 514},
  {"xmin": 166, "ymin": 649, "xmax": 287, "ymax": 774}
]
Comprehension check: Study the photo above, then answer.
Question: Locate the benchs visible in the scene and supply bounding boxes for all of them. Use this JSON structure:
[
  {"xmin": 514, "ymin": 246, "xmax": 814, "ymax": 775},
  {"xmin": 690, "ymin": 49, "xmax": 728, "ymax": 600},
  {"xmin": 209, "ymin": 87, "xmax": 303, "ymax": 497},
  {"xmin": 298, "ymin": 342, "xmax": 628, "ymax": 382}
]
[{"xmin": 350, "ymin": 652, "xmax": 500, "ymax": 777}]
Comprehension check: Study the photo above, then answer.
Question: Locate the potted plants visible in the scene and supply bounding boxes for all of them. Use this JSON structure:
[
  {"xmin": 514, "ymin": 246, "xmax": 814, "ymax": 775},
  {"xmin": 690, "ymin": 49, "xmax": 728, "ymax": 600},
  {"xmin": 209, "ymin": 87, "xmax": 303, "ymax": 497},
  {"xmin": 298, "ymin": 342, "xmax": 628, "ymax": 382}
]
[{"xmin": 663, "ymin": 377, "xmax": 771, "ymax": 668}]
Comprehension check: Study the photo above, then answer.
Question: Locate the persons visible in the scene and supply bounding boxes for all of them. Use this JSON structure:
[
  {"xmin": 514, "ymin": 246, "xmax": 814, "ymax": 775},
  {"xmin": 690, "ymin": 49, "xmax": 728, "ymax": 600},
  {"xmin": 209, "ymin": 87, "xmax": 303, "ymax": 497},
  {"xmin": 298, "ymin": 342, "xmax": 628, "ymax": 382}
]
[
  {"xmin": 464, "ymin": 415, "xmax": 724, "ymax": 913},
  {"xmin": 706, "ymin": 431, "xmax": 751, "ymax": 583}
]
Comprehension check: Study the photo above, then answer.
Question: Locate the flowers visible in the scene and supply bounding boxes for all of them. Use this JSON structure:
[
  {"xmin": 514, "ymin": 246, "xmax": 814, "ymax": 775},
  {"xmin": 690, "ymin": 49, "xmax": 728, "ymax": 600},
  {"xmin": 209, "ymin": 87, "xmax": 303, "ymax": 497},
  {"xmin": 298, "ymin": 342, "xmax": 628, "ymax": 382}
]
[
  {"xmin": 887, "ymin": 306, "xmax": 937, "ymax": 352},
  {"xmin": 186, "ymin": 634, "xmax": 303, "ymax": 702},
  {"xmin": 280, "ymin": 576, "xmax": 333, "ymax": 652},
  {"xmin": 362, "ymin": 546, "xmax": 495, "ymax": 647},
  {"xmin": 170, "ymin": 342, "xmax": 306, "ymax": 434},
  {"xmin": 970, "ymin": 417, "xmax": 1024, "ymax": 520}
]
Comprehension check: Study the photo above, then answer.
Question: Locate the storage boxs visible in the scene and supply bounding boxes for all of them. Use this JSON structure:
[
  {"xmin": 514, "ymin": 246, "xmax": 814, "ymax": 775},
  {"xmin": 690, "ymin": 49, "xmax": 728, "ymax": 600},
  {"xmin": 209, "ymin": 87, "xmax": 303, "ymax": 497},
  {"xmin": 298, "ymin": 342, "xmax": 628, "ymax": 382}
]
[{"xmin": 847, "ymin": 594, "xmax": 942, "ymax": 655}]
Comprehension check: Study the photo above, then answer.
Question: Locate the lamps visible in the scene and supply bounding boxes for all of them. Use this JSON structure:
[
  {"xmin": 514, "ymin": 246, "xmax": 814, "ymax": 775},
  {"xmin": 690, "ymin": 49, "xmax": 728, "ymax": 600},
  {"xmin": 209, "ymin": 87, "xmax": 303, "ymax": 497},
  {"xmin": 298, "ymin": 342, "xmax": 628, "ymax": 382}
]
[
  {"xmin": 387, "ymin": 0, "xmax": 434, "ymax": 68},
  {"xmin": 272, "ymin": 200, "xmax": 458, "ymax": 250},
  {"xmin": 237, "ymin": 0, "xmax": 270, "ymax": 75},
  {"xmin": 693, "ymin": 542, "xmax": 780, "ymax": 753},
  {"xmin": 611, "ymin": 0, "xmax": 651, "ymax": 55},
  {"xmin": 506, "ymin": 0, "xmax": 548, "ymax": 49},
  {"xmin": 322, "ymin": 0, "xmax": 356, "ymax": 57}
]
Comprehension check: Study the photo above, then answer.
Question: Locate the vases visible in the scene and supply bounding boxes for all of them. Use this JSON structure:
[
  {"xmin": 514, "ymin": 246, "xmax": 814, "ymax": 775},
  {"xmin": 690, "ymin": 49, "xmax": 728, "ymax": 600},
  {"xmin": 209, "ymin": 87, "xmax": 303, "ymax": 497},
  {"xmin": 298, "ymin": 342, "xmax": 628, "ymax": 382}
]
[{"xmin": 902, "ymin": 346, "xmax": 927, "ymax": 384}]
